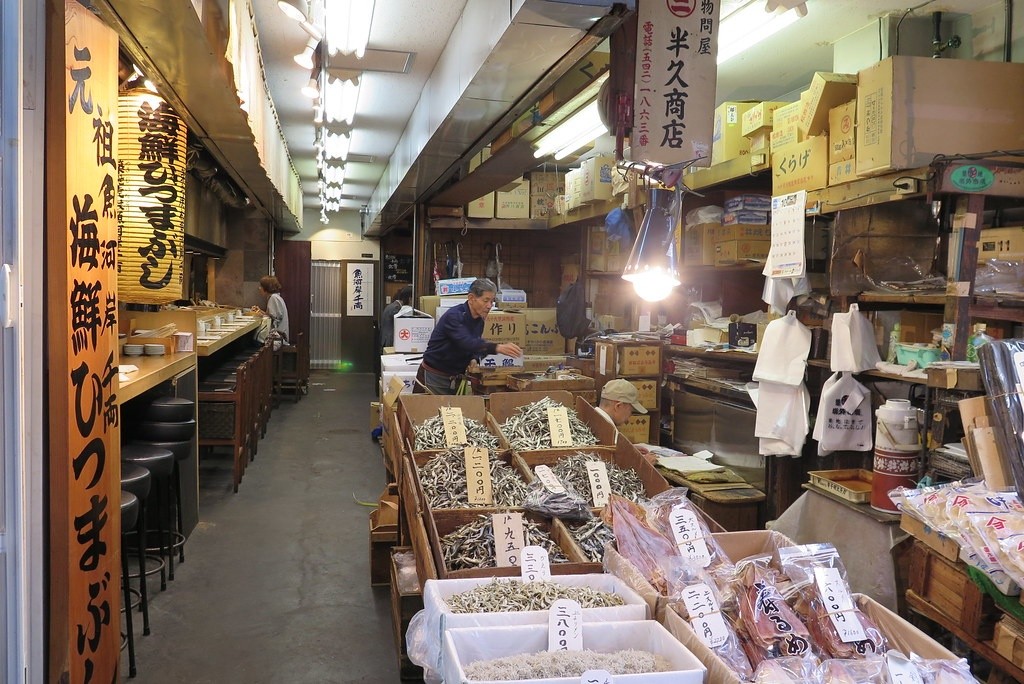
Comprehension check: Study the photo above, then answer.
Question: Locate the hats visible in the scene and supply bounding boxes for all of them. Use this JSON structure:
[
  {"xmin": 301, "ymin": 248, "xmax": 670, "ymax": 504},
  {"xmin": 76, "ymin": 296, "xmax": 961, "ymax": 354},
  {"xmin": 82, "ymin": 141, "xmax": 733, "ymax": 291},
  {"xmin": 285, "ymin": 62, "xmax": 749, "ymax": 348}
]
[
  {"xmin": 601, "ymin": 380, "xmax": 648, "ymax": 414},
  {"xmin": 606, "ymin": 209, "xmax": 630, "ymax": 250}
]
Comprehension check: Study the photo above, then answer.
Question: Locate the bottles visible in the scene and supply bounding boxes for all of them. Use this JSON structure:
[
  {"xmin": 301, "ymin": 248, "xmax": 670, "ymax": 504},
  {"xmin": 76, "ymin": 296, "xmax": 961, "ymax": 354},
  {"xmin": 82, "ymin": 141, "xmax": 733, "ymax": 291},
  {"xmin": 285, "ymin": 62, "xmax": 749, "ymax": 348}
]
[
  {"xmin": 886, "ymin": 322, "xmax": 902, "ymax": 365},
  {"xmin": 966, "ymin": 323, "xmax": 995, "ymax": 364}
]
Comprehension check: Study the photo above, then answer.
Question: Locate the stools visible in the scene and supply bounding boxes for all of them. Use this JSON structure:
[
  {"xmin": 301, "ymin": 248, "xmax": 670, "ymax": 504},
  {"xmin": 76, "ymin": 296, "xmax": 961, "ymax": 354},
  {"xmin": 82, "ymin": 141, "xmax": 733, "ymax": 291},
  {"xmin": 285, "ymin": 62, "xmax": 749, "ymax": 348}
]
[{"xmin": 121, "ymin": 331, "xmax": 305, "ymax": 679}]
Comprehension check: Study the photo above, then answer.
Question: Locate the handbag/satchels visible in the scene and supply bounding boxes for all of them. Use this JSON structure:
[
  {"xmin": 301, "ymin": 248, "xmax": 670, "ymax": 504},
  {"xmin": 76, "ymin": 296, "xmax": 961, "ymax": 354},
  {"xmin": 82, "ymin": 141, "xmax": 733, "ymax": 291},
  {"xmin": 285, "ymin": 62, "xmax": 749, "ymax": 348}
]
[{"xmin": 254, "ymin": 317, "xmax": 272, "ymax": 344}]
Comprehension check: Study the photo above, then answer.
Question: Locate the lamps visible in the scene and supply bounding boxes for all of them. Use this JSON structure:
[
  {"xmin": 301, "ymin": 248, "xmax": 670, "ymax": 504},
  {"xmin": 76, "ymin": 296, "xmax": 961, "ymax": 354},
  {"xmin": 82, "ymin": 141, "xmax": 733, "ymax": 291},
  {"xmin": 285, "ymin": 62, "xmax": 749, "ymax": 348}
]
[
  {"xmin": 278, "ymin": 0, "xmax": 376, "ymax": 226},
  {"xmin": 621, "ymin": 187, "xmax": 682, "ymax": 303},
  {"xmin": 528, "ymin": 0, "xmax": 808, "ymax": 162}
]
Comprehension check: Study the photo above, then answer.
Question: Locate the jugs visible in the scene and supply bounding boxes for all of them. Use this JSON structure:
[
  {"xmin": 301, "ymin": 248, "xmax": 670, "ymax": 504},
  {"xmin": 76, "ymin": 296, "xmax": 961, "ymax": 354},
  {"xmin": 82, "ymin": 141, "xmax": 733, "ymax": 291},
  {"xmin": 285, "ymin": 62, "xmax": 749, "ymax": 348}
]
[{"xmin": 870, "ymin": 398, "xmax": 923, "ymax": 514}]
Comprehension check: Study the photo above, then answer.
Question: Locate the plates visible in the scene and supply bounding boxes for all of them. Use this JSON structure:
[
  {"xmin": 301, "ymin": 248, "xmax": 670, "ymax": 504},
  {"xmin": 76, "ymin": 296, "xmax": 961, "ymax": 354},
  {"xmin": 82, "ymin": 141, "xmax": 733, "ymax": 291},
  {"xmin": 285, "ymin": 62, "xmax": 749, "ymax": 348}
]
[
  {"xmin": 124, "ymin": 344, "xmax": 165, "ymax": 357},
  {"xmin": 118, "ymin": 333, "xmax": 126, "ymax": 337}
]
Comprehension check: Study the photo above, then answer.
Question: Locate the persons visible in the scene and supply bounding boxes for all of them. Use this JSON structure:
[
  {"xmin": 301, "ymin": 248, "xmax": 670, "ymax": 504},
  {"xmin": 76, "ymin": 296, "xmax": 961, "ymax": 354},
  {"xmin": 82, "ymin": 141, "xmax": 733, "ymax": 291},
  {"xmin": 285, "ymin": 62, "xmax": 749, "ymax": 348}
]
[
  {"xmin": 251, "ymin": 277, "xmax": 290, "ymax": 378},
  {"xmin": 376, "ymin": 286, "xmax": 415, "ymax": 399},
  {"xmin": 411, "ymin": 278, "xmax": 520, "ymax": 395},
  {"xmin": 595, "ymin": 379, "xmax": 660, "ymax": 467}
]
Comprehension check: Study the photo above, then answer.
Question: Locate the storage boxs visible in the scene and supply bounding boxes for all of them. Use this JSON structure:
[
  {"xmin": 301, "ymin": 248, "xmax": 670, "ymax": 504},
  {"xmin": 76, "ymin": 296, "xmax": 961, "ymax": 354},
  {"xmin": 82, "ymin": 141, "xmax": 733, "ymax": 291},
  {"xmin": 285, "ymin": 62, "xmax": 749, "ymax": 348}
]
[{"xmin": 369, "ymin": 54, "xmax": 1024, "ymax": 684}]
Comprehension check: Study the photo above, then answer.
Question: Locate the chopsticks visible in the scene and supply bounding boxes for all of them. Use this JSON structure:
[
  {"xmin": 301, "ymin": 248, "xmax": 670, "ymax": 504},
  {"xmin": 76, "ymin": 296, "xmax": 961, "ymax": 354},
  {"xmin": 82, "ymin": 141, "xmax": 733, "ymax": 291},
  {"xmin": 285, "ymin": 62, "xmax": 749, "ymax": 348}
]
[{"xmin": 132, "ymin": 322, "xmax": 180, "ymax": 339}]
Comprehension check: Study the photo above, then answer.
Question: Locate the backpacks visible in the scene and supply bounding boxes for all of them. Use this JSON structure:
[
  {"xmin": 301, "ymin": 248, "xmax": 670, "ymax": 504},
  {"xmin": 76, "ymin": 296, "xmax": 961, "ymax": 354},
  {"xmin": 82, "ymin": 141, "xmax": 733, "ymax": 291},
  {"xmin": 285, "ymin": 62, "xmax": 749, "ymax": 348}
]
[{"xmin": 556, "ymin": 281, "xmax": 589, "ymax": 340}]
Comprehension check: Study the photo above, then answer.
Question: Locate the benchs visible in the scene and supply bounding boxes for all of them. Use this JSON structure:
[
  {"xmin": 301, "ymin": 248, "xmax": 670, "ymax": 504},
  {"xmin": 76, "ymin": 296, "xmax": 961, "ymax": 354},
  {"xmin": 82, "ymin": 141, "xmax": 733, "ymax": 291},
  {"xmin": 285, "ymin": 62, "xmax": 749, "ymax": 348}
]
[{"xmin": 634, "ymin": 442, "xmax": 767, "ymax": 532}]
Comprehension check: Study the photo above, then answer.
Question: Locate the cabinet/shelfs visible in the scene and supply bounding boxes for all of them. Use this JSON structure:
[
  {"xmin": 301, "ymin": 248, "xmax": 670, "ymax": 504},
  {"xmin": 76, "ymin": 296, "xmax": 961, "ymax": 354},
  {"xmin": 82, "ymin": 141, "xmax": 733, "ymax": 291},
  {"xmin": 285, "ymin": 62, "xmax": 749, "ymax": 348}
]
[
  {"xmin": 592, "ymin": 336, "xmax": 665, "ymax": 447},
  {"xmin": 764, "ymin": 162, "xmax": 1024, "ymax": 541}
]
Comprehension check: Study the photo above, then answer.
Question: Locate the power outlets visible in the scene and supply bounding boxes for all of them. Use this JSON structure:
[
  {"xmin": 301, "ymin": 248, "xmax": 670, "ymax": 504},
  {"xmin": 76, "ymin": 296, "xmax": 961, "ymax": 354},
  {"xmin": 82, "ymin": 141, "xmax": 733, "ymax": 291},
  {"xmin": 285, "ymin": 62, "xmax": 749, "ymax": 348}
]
[{"xmin": 897, "ymin": 178, "xmax": 917, "ymax": 195}]
